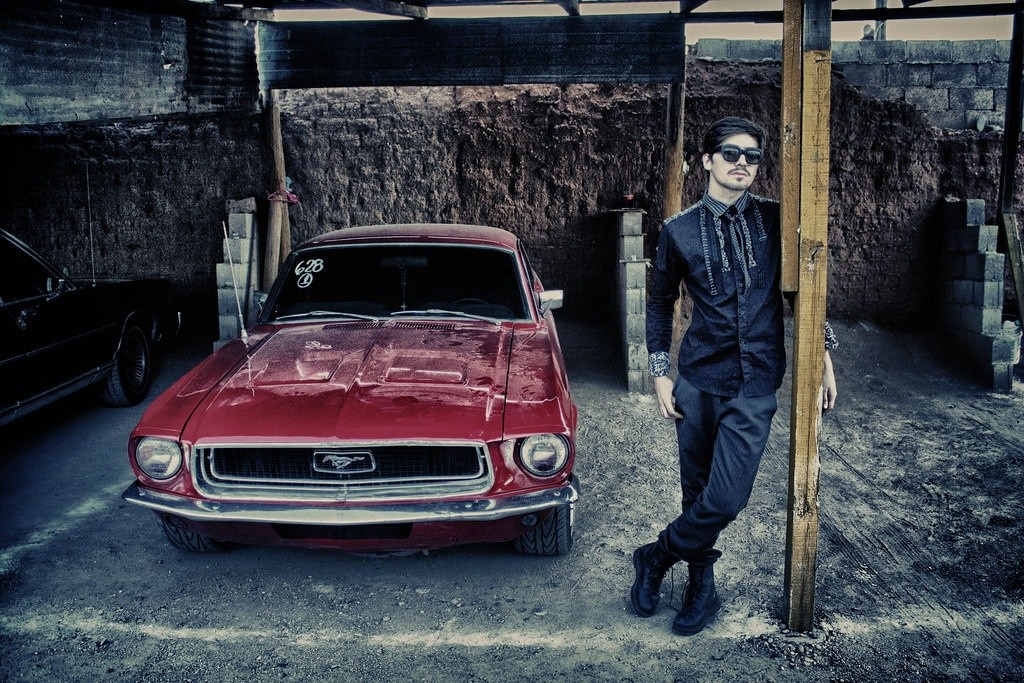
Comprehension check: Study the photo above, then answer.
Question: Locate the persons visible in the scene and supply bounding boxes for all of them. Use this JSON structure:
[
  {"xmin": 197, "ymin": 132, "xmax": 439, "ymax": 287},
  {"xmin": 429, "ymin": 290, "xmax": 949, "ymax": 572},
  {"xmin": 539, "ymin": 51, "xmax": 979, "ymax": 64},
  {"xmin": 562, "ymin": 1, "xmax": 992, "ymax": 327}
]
[{"xmin": 627, "ymin": 114, "xmax": 841, "ymax": 640}]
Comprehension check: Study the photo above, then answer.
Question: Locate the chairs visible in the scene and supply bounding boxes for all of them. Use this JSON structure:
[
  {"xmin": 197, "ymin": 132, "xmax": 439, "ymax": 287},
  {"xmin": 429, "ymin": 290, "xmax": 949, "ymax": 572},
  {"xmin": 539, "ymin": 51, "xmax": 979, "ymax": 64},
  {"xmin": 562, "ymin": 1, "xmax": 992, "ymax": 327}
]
[{"xmin": 299, "ymin": 267, "xmax": 524, "ymax": 326}]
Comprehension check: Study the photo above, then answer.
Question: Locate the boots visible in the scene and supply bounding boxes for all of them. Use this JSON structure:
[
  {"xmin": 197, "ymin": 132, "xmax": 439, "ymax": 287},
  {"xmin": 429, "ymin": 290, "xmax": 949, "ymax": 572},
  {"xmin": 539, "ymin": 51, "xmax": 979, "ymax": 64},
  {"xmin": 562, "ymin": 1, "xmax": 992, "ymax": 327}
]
[
  {"xmin": 629, "ymin": 540, "xmax": 682, "ymax": 616},
  {"xmin": 673, "ymin": 559, "xmax": 721, "ymax": 635}
]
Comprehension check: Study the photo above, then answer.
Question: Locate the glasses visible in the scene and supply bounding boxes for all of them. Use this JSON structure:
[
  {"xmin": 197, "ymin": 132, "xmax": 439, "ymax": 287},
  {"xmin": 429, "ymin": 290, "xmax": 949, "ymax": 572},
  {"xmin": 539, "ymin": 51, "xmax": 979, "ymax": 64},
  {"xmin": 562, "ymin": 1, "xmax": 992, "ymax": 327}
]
[{"xmin": 711, "ymin": 144, "xmax": 765, "ymax": 165}]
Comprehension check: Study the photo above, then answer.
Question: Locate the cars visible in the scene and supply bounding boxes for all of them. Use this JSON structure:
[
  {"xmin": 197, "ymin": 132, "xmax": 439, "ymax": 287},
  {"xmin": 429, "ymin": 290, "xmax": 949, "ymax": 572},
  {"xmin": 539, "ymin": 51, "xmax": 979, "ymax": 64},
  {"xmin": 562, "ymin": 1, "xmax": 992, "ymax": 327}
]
[
  {"xmin": 124, "ymin": 225, "xmax": 579, "ymax": 558},
  {"xmin": 0, "ymin": 230, "xmax": 186, "ymax": 424}
]
